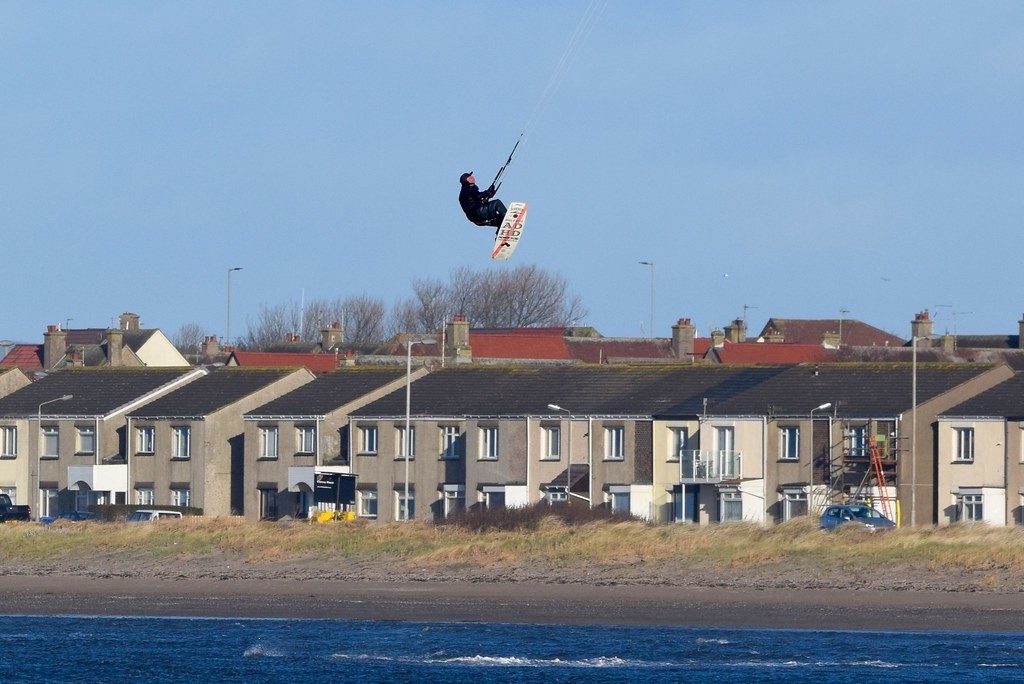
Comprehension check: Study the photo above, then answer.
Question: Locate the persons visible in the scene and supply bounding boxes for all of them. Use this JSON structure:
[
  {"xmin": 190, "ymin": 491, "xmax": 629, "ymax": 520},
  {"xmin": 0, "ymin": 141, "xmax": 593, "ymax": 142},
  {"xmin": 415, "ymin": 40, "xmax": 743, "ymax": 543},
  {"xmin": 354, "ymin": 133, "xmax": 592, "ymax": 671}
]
[{"xmin": 458, "ymin": 171, "xmax": 507, "ymax": 241}]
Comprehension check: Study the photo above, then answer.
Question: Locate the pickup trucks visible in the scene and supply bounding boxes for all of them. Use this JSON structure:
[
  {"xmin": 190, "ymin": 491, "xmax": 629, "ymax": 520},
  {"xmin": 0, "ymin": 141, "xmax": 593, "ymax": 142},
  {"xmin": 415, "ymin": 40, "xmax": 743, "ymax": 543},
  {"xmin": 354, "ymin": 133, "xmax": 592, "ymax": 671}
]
[{"xmin": 0, "ymin": 494, "xmax": 31, "ymax": 522}]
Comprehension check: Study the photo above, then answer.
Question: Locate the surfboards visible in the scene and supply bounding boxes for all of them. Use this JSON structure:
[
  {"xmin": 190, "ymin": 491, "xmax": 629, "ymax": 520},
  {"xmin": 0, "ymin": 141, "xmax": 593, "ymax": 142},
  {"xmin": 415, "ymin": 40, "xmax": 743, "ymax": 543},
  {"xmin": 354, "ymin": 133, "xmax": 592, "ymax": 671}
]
[{"xmin": 491, "ymin": 202, "xmax": 527, "ymax": 260}]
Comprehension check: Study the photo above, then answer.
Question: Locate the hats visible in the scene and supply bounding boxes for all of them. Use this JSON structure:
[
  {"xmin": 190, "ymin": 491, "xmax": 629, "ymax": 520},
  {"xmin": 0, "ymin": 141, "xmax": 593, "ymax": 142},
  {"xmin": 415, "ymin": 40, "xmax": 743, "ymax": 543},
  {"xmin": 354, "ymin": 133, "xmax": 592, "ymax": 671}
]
[{"xmin": 460, "ymin": 171, "xmax": 473, "ymax": 185}]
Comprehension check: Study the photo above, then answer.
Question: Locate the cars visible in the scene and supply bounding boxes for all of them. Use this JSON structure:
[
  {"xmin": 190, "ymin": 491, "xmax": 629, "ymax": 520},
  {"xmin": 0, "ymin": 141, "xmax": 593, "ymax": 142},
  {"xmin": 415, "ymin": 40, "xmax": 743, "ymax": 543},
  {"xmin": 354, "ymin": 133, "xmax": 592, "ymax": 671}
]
[
  {"xmin": 130, "ymin": 510, "xmax": 182, "ymax": 522},
  {"xmin": 819, "ymin": 505, "xmax": 897, "ymax": 532},
  {"xmin": 39, "ymin": 511, "xmax": 95, "ymax": 524}
]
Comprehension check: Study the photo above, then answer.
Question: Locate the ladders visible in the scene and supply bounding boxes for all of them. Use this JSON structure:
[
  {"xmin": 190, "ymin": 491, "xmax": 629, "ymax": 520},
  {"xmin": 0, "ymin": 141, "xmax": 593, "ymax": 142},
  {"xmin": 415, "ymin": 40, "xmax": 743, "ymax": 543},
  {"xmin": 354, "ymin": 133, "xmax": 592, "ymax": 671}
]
[{"xmin": 852, "ymin": 436, "xmax": 894, "ymax": 522}]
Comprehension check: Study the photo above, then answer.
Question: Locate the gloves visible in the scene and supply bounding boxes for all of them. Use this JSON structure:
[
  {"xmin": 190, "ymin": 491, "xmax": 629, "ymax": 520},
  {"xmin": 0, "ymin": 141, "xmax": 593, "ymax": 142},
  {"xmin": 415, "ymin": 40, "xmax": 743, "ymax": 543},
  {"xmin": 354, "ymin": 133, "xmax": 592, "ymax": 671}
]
[{"xmin": 488, "ymin": 185, "xmax": 496, "ymax": 198}]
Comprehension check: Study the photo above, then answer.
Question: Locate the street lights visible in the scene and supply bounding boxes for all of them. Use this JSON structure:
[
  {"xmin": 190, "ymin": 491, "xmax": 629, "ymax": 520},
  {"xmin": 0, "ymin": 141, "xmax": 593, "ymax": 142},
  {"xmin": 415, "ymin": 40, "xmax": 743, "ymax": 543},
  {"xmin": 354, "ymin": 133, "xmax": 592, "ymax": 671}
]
[
  {"xmin": 404, "ymin": 339, "xmax": 437, "ymax": 519},
  {"xmin": 840, "ymin": 310, "xmax": 850, "ymax": 343},
  {"xmin": 227, "ymin": 268, "xmax": 244, "ymax": 347},
  {"xmin": 548, "ymin": 404, "xmax": 571, "ymax": 501},
  {"xmin": 639, "ymin": 262, "xmax": 653, "ymax": 338},
  {"xmin": 911, "ymin": 334, "xmax": 942, "ymax": 525},
  {"xmin": 36, "ymin": 394, "xmax": 73, "ymax": 522},
  {"xmin": 810, "ymin": 403, "xmax": 832, "ymax": 515}
]
